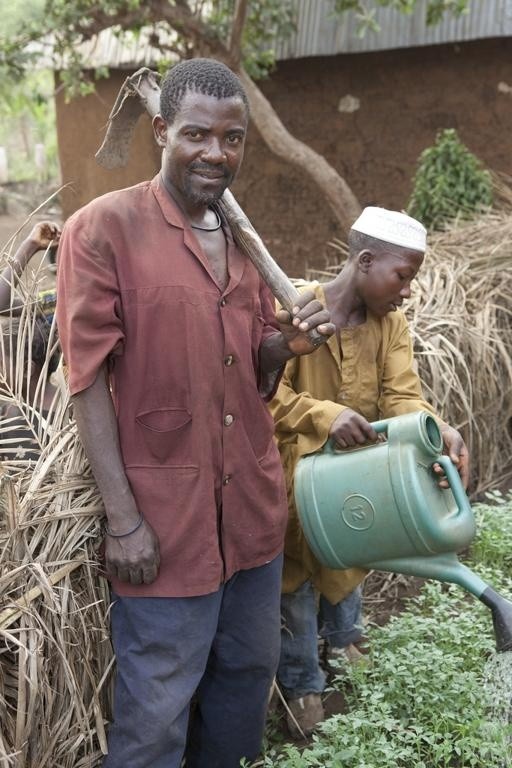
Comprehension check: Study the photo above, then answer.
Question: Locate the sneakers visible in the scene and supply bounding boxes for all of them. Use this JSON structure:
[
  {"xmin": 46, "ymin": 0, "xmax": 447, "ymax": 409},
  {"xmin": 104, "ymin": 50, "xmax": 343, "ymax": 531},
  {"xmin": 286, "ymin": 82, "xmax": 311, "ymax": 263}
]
[
  {"xmin": 322, "ymin": 645, "xmax": 366, "ymax": 682},
  {"xmin": 288, "ymin": 693, "xmax": 324, "ymax": 738}
]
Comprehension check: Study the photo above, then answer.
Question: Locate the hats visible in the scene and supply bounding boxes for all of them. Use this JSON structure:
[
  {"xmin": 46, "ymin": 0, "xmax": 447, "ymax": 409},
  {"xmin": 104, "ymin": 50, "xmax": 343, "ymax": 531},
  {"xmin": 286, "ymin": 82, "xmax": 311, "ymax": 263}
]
[{"xmin": 351, "ymin": 206, "xmax": 427, "ymax": 253}]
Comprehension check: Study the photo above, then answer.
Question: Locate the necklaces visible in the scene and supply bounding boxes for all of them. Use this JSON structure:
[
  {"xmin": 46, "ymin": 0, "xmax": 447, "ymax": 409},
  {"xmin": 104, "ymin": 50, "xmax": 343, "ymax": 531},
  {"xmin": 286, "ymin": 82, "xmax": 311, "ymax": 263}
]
[{"xmin": 190, "ymin": 204, "xmax": 224, "ymax": 232}]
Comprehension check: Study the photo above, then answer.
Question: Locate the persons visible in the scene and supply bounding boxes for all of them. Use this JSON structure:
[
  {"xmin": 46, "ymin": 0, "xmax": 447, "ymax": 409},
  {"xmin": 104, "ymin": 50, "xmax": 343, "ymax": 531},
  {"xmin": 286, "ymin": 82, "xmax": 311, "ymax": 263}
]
[
  {"xmin": 1, "ymin": 217, "xmax": 59, "ymax": 374},
  {"xmin": 264, "ymin": 204, "xmax": 469, "ymax": 738},
  {"xmin": 2, "ymin": 326, "xmax": 70, "ymax": 463},
  {"xmin": 58, "ymin": 57, "xmax": 335, "ymax": 767}
]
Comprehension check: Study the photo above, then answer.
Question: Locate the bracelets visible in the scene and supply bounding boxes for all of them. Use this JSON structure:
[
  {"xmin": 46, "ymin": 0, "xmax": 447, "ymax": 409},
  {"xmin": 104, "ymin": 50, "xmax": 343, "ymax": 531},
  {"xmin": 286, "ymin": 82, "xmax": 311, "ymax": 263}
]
[{"xmin": 105, "ymin": 506, "xmax": 144, "ymax": 536}]
